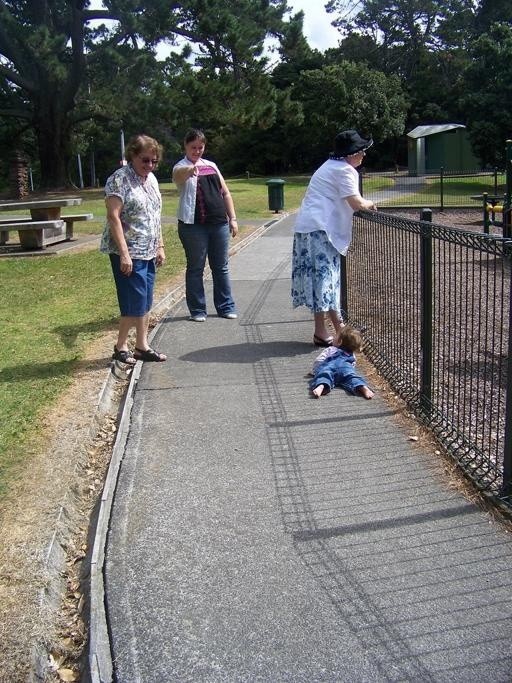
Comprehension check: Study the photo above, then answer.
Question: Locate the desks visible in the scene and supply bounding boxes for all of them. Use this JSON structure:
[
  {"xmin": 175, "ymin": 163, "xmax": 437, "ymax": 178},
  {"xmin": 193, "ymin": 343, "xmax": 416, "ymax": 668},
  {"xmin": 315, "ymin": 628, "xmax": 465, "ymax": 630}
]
[{"xmin": 0, "ymin": 196, "xmax": 84, "ymax": 249}]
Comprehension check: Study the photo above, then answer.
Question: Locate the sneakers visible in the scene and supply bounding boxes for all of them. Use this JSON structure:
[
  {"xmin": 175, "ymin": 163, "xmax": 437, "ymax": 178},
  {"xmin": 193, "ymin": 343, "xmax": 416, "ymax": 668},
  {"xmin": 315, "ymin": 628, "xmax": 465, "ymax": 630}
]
[
  {"xmin": 226, "ymin": 312, "xmax": 238, "ymax": 319},
  {"xmin": 193, "ymin": 315, "xmax": 207, "ymax": 322}
]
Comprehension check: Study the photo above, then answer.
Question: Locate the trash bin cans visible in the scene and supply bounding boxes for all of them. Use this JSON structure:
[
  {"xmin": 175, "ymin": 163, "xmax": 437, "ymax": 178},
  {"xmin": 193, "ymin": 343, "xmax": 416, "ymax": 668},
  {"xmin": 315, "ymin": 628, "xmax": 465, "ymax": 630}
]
[{"xmin": 266, "ymin": 179, "xmax": 286, "ymax": 213}]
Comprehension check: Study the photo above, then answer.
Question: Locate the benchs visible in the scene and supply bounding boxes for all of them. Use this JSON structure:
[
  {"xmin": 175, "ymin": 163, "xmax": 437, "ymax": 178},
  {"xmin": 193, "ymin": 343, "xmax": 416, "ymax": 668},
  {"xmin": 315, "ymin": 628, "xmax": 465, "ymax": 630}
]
[
  {"xmin": 0, "ymin": 217, "xmax": 67, "ymax": 250},
  {"xmin": 0, "ymin": 211, "xmax": 96, "ymax": 243}
]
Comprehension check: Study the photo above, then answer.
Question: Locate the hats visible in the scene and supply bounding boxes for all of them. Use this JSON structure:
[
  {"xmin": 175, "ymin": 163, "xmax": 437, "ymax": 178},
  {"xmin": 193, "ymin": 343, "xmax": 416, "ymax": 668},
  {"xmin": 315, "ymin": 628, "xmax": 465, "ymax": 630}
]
[{"xmin": 328, "ymin": 128, "xmax": 374, "ymax": 158}]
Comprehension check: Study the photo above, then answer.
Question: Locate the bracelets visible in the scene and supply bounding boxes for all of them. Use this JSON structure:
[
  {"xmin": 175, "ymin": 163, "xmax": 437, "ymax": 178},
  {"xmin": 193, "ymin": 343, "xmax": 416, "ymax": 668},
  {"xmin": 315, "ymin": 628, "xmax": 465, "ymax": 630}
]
[
  {"xmin": 156, "ymin": 244, "xmax": 164, "ymax": 249},
  {"xmin": 230, "ymin": 216, "xmax": 237, "ymax": 220}
]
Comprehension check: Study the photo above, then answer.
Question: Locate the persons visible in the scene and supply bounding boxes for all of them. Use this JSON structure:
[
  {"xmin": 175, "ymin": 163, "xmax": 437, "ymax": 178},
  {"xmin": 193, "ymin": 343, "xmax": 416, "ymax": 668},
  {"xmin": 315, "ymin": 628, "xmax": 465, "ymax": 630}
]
[
  {"xmin": 99, "ymin": 134, "xmax": 170, "ymax": 365},
  {"xmin": 290, "ymin": 127, "xmax": 377, "ymax": 347},
  {"xmin": 307, "ymin": 325, "xmax": 375, "ymax": 400},
  {"xmin": 171, "ymin": 129, "xmax": 242, "ymax": 322}
]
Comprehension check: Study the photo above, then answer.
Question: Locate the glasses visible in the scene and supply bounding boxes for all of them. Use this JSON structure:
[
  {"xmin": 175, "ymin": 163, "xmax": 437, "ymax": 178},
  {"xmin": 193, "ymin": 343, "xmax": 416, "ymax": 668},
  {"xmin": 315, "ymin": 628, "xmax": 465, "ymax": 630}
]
[{"xmin": 134, "ymin": 150, "xmax": 160, "ymax": 163}]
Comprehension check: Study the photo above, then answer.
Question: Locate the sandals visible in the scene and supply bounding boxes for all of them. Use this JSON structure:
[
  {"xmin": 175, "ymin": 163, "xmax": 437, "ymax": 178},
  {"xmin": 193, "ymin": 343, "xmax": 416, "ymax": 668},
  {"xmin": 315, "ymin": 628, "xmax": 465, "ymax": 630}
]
[
  {"xmin": 312, "ymin": 332, "xmax": 334, "ymax": 347},
  {"xmin": 133, "ymin": 343, "xmax": 169, "ymax": 363},
  {"xmin": 112, "ymin": 343, "xmax": 137, "ymax": 364}
]
